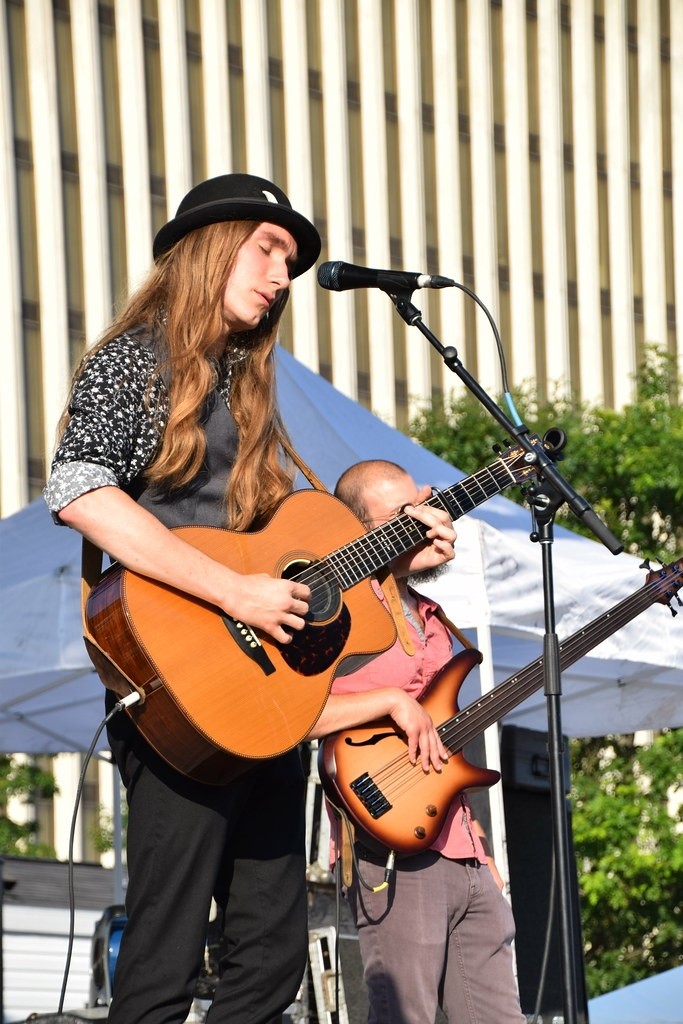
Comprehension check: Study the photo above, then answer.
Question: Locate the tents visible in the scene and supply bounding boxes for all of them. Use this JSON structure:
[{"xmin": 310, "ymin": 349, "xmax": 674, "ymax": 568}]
[{"xmin": 0, "ymin": 338, "xmax": 683, "ymax": 990}]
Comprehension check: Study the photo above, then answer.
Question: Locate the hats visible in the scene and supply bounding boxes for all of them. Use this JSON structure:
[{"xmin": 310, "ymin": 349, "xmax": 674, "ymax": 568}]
[{"xmin": 153, "ymin": 176, "xmax": 323, "ymax": 280}]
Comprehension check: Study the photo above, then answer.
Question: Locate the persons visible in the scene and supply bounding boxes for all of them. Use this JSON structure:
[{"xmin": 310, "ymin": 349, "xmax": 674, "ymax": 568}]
[
  {"xmin": 45, "ymin": 172, "xmax": 455, "ymax": 1024},
  {"xmin": 299, "ymin": 461, "xmax": 528, "ymax": 1024}
]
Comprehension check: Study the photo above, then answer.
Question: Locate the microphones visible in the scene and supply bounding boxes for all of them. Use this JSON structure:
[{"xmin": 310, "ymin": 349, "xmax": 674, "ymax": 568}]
[{"xmin": 318, "ymin": 261, "xmax": 455, "ymax": 292}]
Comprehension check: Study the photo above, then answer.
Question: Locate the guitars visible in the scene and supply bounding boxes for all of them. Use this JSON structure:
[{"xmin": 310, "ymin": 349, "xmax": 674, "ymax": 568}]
[
  {"xmin": 317, "ymin": 557, "xmax": 682, "ymax": 856},
  {"xmin": 84, "ymin": 426, "xmax": 565, "ymax": 785}
]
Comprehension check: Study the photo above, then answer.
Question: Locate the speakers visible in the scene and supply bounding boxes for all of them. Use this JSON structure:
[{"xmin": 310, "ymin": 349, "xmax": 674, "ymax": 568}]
[{"xmin": 502, "ymin": 785, "xmax": 589, "ymax": 1024}]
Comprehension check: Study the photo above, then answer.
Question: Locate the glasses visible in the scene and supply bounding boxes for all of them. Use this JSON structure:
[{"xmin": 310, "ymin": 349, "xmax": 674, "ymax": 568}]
[{"xmin": 361, "ymin": 503, "xmax": 414, "ymax": 523}]
